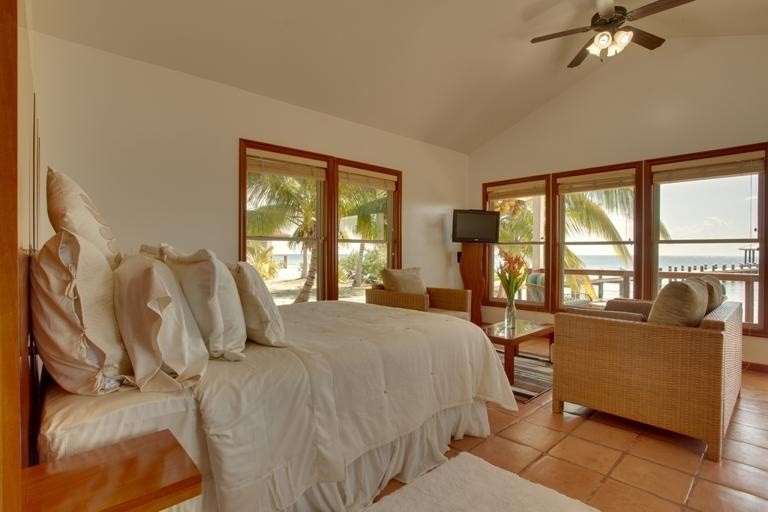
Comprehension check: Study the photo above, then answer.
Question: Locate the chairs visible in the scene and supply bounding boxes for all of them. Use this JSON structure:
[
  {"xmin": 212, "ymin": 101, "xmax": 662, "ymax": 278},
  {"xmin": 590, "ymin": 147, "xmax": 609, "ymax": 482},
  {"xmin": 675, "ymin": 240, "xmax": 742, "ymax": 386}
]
[
  {"xmin": 552, "ymin": 298, "xmax": 742, "ymax": 463},
  {"xmin": 365, "ymin": 284, "xmax": 471, "ymax": 324}
]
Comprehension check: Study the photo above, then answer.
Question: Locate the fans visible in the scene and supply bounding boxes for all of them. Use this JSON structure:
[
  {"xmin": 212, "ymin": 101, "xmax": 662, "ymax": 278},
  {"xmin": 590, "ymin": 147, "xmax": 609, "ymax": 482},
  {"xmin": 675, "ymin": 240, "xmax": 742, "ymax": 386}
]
[{"xmin": 528, "ymin": 0, "xmax": 696, "ymax": 67}]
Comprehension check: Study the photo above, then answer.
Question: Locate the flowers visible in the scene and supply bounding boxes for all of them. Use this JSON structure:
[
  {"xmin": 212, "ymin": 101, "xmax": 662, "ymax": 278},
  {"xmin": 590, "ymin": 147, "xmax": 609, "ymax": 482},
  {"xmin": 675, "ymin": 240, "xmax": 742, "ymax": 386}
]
[{"xmin": 496, "ymin": 250, "xmax": 528, "ymax": 324}]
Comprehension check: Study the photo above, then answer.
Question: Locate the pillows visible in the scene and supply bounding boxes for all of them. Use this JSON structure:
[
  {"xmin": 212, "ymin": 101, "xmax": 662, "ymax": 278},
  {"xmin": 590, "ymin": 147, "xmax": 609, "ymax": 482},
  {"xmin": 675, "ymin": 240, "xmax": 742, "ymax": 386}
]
[
  {"xmin": 685, "ymin": 274, "xmax": 721, "ymax": 311},
  {"xmin": 226, "ymin": 260, "xmax": 295, "ymax": 347},
  {"xmin": 32, "ymin": 229, "xmax": 132, "ymax": 394},
  {"xmin": 646, "ymin": 280, "xmax": 708, "ymax": 327},
  {"xmin": 161, "ymin": 244, "xmax": 249, "ymax": 360},
  {"xmin": 113, "ymin": 252, "xmax": 209, "ymax": 391},
  {"xmin": 47, "ymin": 166, "xmax": 124, "ymax": 269},
  {"xmin": 379, "ymin": 268, "xmax": 428, "ymax": 294}
]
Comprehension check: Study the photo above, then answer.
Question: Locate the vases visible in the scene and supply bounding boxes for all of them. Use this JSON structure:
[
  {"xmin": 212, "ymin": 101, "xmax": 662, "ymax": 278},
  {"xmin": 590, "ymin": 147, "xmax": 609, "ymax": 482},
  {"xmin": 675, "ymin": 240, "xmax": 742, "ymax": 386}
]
[{"xmin": 504, "ymin": 299, "xmax": 517, "ymax": 330}]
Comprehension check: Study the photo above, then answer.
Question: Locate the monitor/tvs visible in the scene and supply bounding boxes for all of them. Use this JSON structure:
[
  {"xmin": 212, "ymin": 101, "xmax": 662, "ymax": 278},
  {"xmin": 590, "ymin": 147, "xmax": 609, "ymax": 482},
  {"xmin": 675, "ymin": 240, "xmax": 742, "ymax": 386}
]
[{"xmin": 452, "ymin": 209, "xmax": 501, "ymax": 244}]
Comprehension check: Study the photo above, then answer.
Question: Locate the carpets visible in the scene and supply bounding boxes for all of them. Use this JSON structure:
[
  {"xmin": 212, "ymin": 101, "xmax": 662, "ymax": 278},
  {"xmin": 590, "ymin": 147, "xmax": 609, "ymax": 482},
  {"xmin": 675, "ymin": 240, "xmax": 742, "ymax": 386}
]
[
  {"xmin": 364, "ymin": 452, "xmax": 597, "ymax": 511},
  {"xmin": 491, "ymin": 347, "xmax": 553, "ymax": 403}
]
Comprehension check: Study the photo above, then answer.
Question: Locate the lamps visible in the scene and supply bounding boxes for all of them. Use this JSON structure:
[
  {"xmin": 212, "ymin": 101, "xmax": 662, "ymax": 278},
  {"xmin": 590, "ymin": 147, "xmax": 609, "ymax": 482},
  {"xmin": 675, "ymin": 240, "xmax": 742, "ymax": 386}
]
[{"xmin": 586, "ymin": 4, "xmax": 632, "ymax": 59}]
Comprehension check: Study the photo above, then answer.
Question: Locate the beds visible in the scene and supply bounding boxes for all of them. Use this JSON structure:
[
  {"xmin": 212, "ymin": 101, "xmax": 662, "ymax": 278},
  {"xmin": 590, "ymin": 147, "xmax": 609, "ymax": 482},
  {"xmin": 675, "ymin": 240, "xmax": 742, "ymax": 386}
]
[{"xmin": 37, "ymin": 300, "xmax": 517, "ymax": 511}]
